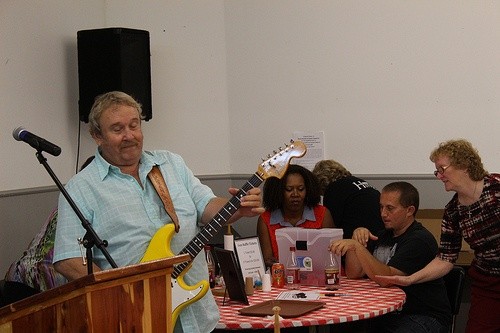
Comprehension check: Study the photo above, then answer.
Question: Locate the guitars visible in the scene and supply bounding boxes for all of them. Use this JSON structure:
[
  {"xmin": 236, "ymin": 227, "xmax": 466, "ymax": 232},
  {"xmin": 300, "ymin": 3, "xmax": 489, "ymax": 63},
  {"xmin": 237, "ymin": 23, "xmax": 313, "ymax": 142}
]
[{"xmin": 138, "ymin": 138, "xmax": 308, "ymax": 333}]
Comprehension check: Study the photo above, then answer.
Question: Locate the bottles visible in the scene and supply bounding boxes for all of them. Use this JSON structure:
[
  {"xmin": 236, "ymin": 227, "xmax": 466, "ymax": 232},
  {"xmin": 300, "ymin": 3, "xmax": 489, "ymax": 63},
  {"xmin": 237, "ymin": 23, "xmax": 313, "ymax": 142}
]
[
  {"xmin": 323, "ymin": 245, "xmax": 340, "ymax": 296},
  {"xmin": 286, "ymin": 247, "xmax": 300, "ymax": 290}
]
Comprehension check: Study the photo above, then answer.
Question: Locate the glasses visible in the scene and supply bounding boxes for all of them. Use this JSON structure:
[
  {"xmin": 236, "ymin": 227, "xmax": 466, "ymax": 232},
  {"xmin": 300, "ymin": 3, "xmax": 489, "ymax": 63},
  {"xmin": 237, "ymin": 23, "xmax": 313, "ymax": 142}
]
[{"xmin": 434, "ymin": 161, "xmax": 458, "ymax": 176}]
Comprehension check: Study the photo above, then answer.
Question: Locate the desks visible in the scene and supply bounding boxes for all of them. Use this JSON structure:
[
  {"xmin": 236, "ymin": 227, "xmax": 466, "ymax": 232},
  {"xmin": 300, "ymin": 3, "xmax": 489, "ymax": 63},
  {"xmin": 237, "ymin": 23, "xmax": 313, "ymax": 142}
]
[{"xmin": 211, "ymin": 276, "xmax": 406, "ymax": 331}]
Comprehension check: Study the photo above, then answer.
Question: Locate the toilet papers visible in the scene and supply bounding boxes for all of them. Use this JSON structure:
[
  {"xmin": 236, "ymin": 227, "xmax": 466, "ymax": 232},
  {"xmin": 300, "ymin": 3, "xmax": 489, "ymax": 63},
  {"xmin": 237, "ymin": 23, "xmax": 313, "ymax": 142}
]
[{"xmin": 261, "ymin": 272, "xmax": 273, "ymax": 292}]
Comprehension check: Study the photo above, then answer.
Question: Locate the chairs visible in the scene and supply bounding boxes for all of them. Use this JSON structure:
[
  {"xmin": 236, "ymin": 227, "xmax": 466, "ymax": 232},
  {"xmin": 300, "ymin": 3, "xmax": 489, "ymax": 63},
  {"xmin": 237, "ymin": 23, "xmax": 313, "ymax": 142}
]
[{"xmin": 427, "ymin": 267, "xmax": 464, "ymax": 333}]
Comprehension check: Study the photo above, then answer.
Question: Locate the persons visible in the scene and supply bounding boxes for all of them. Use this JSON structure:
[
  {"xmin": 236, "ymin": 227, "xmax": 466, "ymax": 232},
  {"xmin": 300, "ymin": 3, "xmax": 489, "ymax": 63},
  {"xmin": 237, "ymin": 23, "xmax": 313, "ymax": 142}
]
[
  {"xmin": 257, "ymin": 165, "xmax": 333, "ymax": 273},
  {"xmin": 52, "ymin": 92, "xmax": 265, "ymax": 333},
  {"xmin": 328, "ymin": 182, "xmax": 450, "ymax": 333},
  {"xmin": 376, "ymin": 140, "xmax": 500, "ymax": 333},
  {"xmin": 313, "ymin": 161, "xmax": 395, "ymax": 255},
  {"xmin": 5, "ymin": 155, "xmax": 95, "ymax": 293}
]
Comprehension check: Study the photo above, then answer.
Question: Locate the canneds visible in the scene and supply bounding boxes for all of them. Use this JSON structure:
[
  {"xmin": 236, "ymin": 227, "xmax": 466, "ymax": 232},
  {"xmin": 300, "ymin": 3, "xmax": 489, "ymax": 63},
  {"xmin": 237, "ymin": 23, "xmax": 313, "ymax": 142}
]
[{"xmin": 272, "ymin": 263, "xmax": 285, "ymax": 288}]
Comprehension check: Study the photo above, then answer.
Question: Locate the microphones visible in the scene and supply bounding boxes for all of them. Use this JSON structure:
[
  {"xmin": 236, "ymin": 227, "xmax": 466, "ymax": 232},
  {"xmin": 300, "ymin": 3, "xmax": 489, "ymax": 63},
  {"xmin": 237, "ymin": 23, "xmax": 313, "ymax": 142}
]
[{"xmin": 12, "ymin": 127, "xmax": 61, "ymax": 157}]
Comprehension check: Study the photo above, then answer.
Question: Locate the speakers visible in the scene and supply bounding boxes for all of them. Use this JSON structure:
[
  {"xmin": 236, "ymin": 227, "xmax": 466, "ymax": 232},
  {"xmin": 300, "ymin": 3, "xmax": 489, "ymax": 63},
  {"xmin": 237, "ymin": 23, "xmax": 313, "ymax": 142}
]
[{"xmin": 76, "ymin": 27, "xmax": 152, "ymax": 125}]
